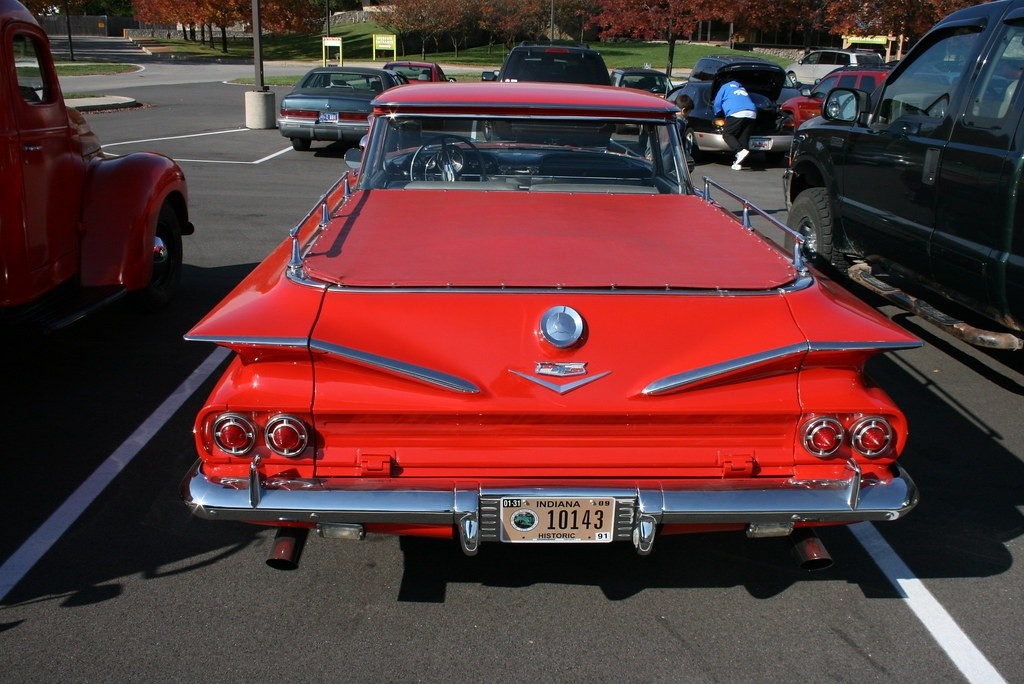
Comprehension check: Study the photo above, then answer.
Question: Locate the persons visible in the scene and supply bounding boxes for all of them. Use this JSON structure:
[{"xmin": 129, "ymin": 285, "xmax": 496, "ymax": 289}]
[
  {"xmin": 714, "ymin": 78, "xmax": 757, "ymax": 170},
  {"xmin": 645, "ymin": 95, "xmax": 694, "ymax": 171}
]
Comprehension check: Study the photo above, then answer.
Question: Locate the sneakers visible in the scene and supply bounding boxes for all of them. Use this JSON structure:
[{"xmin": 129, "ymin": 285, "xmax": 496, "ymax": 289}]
[
  {"xmin": 731, "ymin": 161, "xmax": 741, "ymax": 171},
  {"xmin": 735, "ymin": 149, "xmax": 750, "ymax": 164}
]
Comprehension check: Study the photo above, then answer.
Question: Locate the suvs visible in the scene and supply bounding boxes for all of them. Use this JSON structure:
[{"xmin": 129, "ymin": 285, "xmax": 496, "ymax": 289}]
[
  {"xmin": 782, "ymin": 49, "xmax": 886, "ymax": 86},
  {"xmin": 778, "ymin": 60, "xmax": 904, "ymax": 138},
  {"xmin": 480, "ymin": 41, "xmax": 618, "ymax": 144},
  {"xmin": 781, "ymin": 2, "xmax": 1024, "ymax": 355}
]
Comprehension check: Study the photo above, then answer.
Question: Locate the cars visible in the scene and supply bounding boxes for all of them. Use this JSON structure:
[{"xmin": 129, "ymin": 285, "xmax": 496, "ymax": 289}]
[
  {"xmin": 184, "ymin": 80, "xmax": 927, "ymax": 572},
  {"xmin": 611, "ymin": 66, "xmax": 676, "ymax": 134},
  {"xmin": 669, "ymin": 54, "xmax": 809, "ymax": 160},
  {"xmin": 375, "ymin": 61, "xmax": 457, "ymax": 92},
  {"xmin": 278, "ymin": 66, "xmax": 411, "ymax": 150},
  {"xmin": 0, "ymin": 0, "xmax": 195, "ymax": 318}
]
